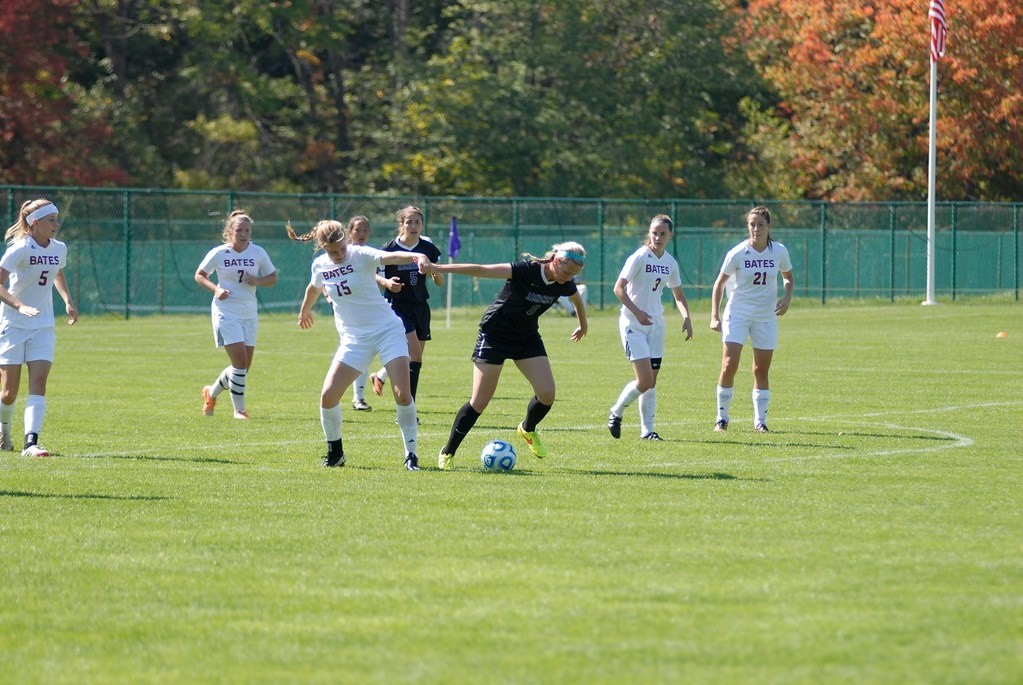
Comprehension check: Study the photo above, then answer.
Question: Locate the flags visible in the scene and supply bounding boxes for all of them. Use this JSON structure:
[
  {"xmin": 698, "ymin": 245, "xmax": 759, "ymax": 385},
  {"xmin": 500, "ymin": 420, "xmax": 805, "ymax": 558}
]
[
  {"xmin": 447, "ymin": 220, "xmax": 461, "ymax": 259},
  {"xmin": 928, "ymin": 0, "xmax": 947, "ymax": 62}
]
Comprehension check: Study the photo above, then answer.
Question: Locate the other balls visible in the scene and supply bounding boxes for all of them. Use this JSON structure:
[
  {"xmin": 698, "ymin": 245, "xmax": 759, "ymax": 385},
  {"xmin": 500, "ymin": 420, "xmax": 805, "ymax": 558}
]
[{"xmin": 997, "ymin": 331, "xmax": 1008, "ymax": 338}]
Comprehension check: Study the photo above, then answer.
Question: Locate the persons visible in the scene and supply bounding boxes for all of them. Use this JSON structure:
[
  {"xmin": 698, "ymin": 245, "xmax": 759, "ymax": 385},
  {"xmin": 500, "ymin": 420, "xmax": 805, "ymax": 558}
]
[
  {"xmin": 558, "ymin": 284, "xmax": 587, "ymax": 316},
  {"xmin": 414, "ymin": 241, "xmax": 588, "ymax": 470},
  {"xmin": 710, "ymin": 206, "xmax": 793, "ymax": 434},
  {"xmin": 609, "ymin": 214, "xmax": 692, "ymax": 441},
  {"xmin": 194, "ymin": 210, "xmax": 277, "ymax": 420},
  {"xmin": 0, "ymin": 198, "xmax": 78, "ymax": 457},
  {"xmin": 349, "ymin": 206, "xmax": 445, "ymax": 425},
  {"xmin": 287, "ymin": 220, "xmax": 434, "ymax": 472}
]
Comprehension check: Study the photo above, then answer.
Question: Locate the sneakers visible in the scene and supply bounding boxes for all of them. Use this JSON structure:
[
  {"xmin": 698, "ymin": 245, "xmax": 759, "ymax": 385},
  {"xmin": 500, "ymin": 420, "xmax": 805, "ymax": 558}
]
[
  {"xmin": 369, "ymin": 371, "xmax": 385, "ymax": 396},
  {"xmin": 396, "ymin": 415, "xmax": 422, "ymax": 425},
  {"xmin": 713, "ymin": 420, "xmax": 729, "ymax": 432},
  {"xmin": 0, "ymin": 432, "xmax": 14, "ymax": 451},
  {"xmin": 21, "ymin": 444, "xmax": 49, "ymax": 457},
  {"xmin": 404, "ymin": 452, "xmax": 420, "ymax": 471},
  {"xmin": 753, "ymin": 423, "xmax": 768, "ymax": 432},
  {"xmin": 437, "ymin": 445, "xmax": 454, "ymax": 470},
  {"xmin": 320, "ymin": 452, "xmax": 346, "ymax": 467},
  {"xmin": 234, "ymin": 409, "xmax": 248, "ymax": 420},
  {"xmin": 202, "ymin": 385, "xmax": 217, "ymax": 417},
  {"xmin": 517, "ymin": 419, "xmax": 547, "ymax": 459},
  {"xmin": 643, "ymin": 431, "xmax": 662, "ymax": 441},
  {"xmin": 352, "ymin": 399, "xmax": 372, "ymax": 411},
  {"xmin": 608, "ymin": 407, "xmax": 623, "ymax": 439}
]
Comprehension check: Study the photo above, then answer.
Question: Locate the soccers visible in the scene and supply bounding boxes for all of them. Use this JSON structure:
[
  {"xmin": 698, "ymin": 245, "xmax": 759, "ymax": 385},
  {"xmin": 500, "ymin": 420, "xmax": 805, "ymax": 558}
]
[{"xmin": 480, "ymin": 439, "xmax": 517, "ymax": 471}]
